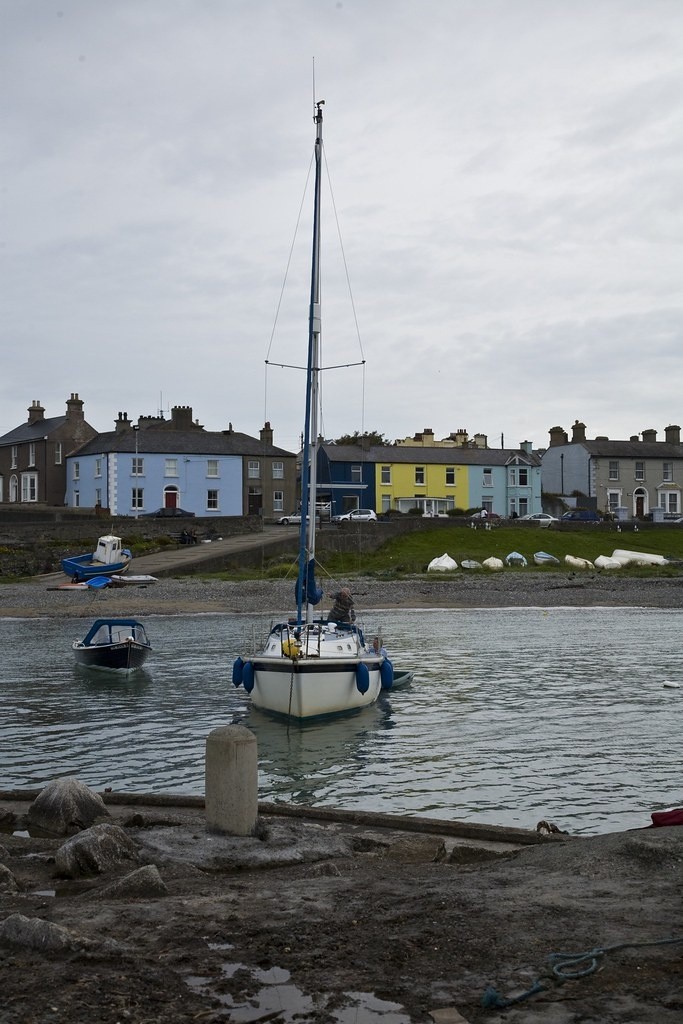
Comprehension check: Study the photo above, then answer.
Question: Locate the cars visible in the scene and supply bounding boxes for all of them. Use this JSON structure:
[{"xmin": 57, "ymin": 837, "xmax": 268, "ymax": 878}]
[
  {"xmin": 514, "ymin": 513, "xmax": 558, "ymax": 528},
  {"xmin": 470, "ymin": 512, "xmax": 501, "ymax": 521},
  {"xmin": 277, "ymin": 511, "xmax": 310, "ymax": 526}
]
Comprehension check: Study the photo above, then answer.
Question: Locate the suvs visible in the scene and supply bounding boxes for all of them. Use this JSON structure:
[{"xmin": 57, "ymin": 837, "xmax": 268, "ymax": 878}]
[
  {"xmin": 558, "ymin": 509, "xmax": 601, "ymax": 521},
  {"xmin": 331, "ymin": 508, "xmax": 377, "ymax": 526}
]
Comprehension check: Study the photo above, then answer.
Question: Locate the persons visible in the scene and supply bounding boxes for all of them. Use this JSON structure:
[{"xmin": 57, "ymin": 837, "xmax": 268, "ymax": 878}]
[
  {"xmin": 511, "ymin": 510, "xmax": 518, "ymax": 519},
  {"xmin": 480, "ymin": 506, "xmax": 488, "ymax": 518},
  {"xmin": 367, "ymin": 636, "xmax": 387, "ymax": 659},
  {"xmin": 324, "ymin": 587, "xmax": 356, "ymax": 624}
]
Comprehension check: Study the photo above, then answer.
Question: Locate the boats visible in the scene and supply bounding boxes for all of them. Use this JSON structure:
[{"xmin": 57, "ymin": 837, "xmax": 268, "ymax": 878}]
[
  {"xmin": 111, "ymin": 574, "xmax": 159, "ymax": 584},
  {"xmin": 58, "ymin": 584, "xmax": 88, "ymax": 590},
  {"xmin": 461, "ymin": 558, "xmax": 483, "ymax": 570},
  {"xmin": 72, "ymin": 619, "xmax": 153, "ymax": 676},
  {"xmin": 391, "ymin": 668, "xmax": 417, "ymax": 690},
  {"xmin": 62, "ymin": 535, "xmax": 133, "ymax": 580},
  {"xmin": 506, "ymin": 551, "xmax": 528, "ymax": 568},
  {"xmin": 484, "ymin": 556, "xmax": 503, "ymax": 570},
  {"xmin": 594, "ymin": 547, "xmax": 667, "ymax": 569},
  {"xmin": 427, "ymin": 553, "xmax": 453, "ymax": 572},
  {"xmin": 86, "ymin": 576, "xmax": 113, "ymax": 590},
  {"xmin": 534, "ymin": 551, "xmax": 560, "ymax": 564},
  {"xmin": 565, "ymin": 553, "xmax": 595, "ymax": 570}
]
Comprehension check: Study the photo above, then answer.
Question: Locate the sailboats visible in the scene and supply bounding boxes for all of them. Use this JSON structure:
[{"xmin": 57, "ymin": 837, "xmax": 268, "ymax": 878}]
[{"xmin": 231, "ymin": 56, "xmax": 396, "ymax": 722}]
